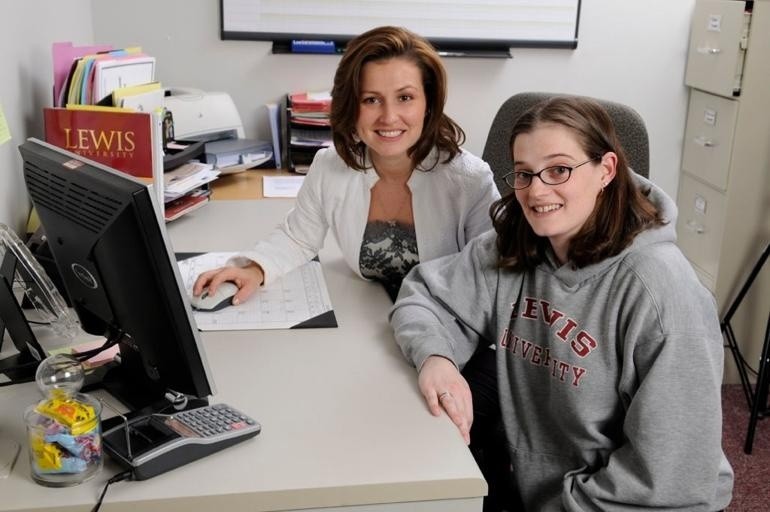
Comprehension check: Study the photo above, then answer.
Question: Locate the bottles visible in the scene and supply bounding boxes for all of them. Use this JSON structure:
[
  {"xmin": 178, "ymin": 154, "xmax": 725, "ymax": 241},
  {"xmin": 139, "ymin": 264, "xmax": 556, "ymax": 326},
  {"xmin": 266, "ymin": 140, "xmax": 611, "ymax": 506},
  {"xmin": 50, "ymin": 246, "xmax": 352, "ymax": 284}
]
[{"xmin": 23, "ymin": 354, "xmax": 103, "ymax": 488}]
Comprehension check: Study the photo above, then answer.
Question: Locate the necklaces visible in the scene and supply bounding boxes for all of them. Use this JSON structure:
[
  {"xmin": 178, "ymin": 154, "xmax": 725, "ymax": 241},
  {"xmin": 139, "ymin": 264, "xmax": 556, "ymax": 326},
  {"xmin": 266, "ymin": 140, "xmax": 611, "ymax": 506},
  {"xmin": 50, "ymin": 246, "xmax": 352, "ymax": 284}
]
[{"xmin": 373, "ymin": 180, "xmax": 411, "ymax": 228}]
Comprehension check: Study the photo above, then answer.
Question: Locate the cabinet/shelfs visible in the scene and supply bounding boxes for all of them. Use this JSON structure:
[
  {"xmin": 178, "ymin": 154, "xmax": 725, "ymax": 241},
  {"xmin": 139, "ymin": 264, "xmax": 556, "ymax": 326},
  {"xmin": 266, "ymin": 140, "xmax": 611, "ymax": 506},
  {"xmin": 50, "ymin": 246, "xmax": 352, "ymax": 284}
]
[{"xmin": 668, "ymin": 0, "xmax": 770, "ymax": 385}]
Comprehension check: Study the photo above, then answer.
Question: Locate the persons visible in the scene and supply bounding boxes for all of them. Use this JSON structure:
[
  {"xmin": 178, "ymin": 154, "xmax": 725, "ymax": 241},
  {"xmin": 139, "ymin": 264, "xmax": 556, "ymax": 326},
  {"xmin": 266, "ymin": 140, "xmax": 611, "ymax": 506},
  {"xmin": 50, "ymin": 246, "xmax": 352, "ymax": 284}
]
[
  {"xmin": 390, "ymin": 94, "xmax": 736, "ymax": 510},
  {"xmin": 189, "ymin": 26, "xmax": 507, "ymax": 323}
]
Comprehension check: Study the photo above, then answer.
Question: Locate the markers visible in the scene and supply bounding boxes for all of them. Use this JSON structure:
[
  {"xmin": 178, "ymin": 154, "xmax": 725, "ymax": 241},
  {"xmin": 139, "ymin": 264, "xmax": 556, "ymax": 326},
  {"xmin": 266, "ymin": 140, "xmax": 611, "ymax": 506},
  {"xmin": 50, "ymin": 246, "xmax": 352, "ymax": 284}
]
[
  {"xmin": 437, "ymin": 51, "xmax": 468, "ymax": 57},
  {"xmin": 336, "ymin": 48, "xmax": 347, "ymax": 53}
]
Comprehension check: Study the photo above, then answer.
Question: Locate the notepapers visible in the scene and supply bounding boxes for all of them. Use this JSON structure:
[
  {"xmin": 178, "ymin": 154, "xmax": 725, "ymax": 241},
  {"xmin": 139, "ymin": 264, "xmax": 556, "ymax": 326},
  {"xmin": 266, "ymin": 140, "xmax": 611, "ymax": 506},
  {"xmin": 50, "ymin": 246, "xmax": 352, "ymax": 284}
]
[{"xmin": 48, "ymin": 340, "xmax": 119, "ymax": 374}]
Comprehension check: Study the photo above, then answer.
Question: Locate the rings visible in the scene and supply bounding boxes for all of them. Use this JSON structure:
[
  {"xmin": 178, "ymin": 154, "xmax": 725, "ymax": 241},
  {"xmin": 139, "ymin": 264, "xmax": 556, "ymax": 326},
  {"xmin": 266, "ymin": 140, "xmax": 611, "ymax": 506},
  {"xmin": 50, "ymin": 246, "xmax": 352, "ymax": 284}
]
[{"xmin": 438, "ymin": 391, "xmax": 452, "ymax": 401}]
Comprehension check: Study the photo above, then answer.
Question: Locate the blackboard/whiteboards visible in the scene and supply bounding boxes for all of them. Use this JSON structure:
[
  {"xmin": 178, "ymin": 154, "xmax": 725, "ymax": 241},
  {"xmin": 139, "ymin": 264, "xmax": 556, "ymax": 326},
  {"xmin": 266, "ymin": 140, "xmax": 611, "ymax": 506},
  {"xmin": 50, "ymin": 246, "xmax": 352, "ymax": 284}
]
[{"xmin": 220, "ymin": 0, "xmax": 581, "ymax": 58}]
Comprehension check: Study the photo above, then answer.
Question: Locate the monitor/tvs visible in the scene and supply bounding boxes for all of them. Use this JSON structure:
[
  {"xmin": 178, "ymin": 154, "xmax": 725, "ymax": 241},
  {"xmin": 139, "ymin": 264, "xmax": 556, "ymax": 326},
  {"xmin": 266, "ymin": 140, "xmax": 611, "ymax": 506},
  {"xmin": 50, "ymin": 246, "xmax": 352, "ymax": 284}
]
[{"xmin": 19, "ymin": 137, "xmax": 217, "ymax": 413}]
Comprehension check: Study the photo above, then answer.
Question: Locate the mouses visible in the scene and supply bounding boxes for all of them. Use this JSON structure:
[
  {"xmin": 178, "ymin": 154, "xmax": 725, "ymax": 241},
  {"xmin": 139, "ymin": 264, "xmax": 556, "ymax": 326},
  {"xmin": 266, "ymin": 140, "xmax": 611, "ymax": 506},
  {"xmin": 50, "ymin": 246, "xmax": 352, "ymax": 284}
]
[{"xmin": 190, "ymin": 282, "xmax": 239, "ymax": 312}]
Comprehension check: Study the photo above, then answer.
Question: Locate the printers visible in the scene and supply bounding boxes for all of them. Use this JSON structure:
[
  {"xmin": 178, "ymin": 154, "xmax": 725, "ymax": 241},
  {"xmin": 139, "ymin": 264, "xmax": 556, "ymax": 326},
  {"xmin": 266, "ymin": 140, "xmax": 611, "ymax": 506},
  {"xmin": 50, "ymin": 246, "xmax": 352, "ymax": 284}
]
[{"xmin": 118, "ymin": 87, "xmax": 274, "ymax": 175}]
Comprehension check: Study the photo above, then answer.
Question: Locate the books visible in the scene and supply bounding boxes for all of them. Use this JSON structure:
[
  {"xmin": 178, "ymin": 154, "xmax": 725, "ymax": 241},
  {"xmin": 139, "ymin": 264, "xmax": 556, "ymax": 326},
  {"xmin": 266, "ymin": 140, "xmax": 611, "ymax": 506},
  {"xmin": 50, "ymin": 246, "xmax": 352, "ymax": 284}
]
[
  {"xmin": 287, "ymin": 87, "xmax": 335, "ymax": 177},
  {"xmin": 42, "ymin": 38, "xmax": 221, "ymax": 233}
]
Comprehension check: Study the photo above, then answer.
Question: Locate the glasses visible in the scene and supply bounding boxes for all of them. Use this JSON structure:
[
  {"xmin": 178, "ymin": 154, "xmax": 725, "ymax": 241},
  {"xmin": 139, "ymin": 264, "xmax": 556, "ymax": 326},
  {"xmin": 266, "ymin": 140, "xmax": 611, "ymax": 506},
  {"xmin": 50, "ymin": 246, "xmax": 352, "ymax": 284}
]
[{"xmin": 501, "ymin": 159, "xmax": 593, "ymax": 191}]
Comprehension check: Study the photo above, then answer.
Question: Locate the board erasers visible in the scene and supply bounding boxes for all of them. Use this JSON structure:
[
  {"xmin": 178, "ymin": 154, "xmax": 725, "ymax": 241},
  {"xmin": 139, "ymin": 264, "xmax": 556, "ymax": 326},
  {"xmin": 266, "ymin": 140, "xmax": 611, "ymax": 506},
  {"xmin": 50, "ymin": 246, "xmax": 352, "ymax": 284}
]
[{"xmin": 291, "ymin": 40, "xmax": 335, "ymax": 53}]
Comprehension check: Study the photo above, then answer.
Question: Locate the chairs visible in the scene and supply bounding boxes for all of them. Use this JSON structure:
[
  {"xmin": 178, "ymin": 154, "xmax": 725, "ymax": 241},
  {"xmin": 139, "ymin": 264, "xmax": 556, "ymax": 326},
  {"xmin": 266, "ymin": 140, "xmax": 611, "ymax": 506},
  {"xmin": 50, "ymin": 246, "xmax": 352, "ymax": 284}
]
[{"xmin": 479, "ymin": 93, "xmax": 650, "ymax": 351}]
